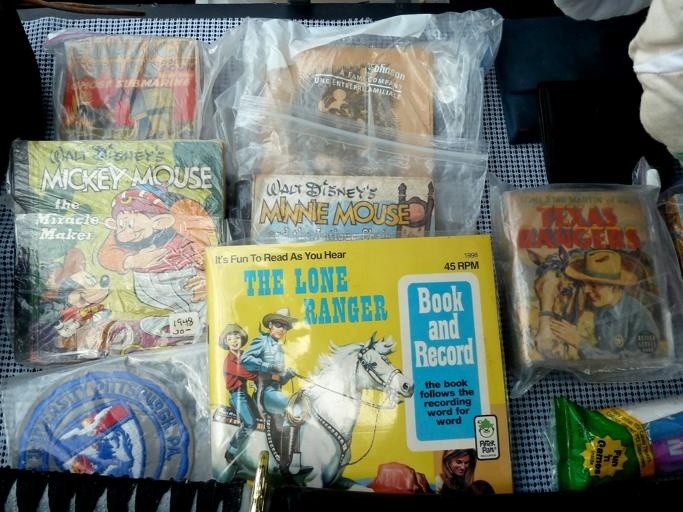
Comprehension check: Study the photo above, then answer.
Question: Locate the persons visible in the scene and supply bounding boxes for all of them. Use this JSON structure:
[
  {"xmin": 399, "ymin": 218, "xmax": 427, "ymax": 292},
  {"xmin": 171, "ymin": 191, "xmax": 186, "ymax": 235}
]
[
  {"xmin": 239, "ymin": 309, "xmax": 313, "ymax": 479},
  {"xmin": 218, "ymin": 324, "xmax": 281, "ymax": 466},
  {"xmin": 434, "ymin": 449, "xmax": 477, "ymax": 493},
  {"xmin": 96, "ymin": 183, "xmax": 217, "ymax": 333},
  {"xmin": 549, "ymin": 249, "xmax": 660, "ymax": 360}
]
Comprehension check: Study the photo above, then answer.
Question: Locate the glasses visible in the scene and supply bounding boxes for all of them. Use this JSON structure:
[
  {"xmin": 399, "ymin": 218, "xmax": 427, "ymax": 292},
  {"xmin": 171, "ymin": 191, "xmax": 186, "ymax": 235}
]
[{"xmin": 275, "ymin": 321, "xmax": 288, "ymax": 329}]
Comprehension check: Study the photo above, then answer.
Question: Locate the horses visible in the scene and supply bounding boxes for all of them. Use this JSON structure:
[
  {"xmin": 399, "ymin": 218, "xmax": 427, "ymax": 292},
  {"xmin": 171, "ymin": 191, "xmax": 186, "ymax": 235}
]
[
  {"xmin": 209, "ymin": 330, "xmax": 415, "ymax": 490},
  {"xmin": 527, "ymin": 245, "xmax": 665, "ymax": 358}
]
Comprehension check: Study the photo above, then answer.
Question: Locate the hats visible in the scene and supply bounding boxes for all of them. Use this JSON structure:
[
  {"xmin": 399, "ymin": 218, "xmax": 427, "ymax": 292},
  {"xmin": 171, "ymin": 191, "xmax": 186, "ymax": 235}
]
[
  {"xmin": 565, "ymin": 250, "xmax": 649, "ymax": 286},
  {"xmin": 262, "ymin": 309, "xmax": 298, "ymax": 330},
  {"xmin": 219, "ymin": 324, "xmax": 247, "ymax": 350},
  {"xmin": 40, "ymin": 249, "xmax": 85, "ymax": 301}
]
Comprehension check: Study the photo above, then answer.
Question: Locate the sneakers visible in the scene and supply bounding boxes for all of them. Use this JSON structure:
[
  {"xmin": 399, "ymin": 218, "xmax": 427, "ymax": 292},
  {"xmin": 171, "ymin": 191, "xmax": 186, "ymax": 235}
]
[
  {"xmin": 225, "ymin": 451, "xmax": 237, "ymax": 465},
  {"xmin": 279, "ymin": 464, "xmax": 313, "ymax": 477}
]
